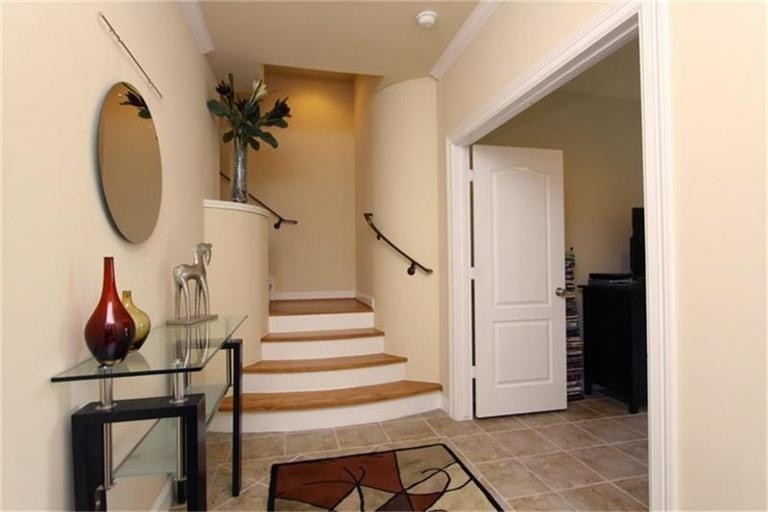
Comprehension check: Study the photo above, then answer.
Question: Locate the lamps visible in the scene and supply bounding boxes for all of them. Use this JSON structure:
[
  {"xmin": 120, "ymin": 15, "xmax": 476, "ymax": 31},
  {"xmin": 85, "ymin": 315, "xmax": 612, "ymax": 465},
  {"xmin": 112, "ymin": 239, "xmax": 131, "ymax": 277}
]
[{"xmin": 415, "ymin": 10, "xmax": 437, "ymax": 31}]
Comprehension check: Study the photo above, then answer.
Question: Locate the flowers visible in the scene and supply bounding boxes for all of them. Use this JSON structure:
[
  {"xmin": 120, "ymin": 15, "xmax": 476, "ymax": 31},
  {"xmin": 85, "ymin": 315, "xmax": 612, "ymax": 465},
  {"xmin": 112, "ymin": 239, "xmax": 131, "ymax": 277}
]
[{"xmin": 206, "ymin": 71, "xmax": 293, "ymax": 150}]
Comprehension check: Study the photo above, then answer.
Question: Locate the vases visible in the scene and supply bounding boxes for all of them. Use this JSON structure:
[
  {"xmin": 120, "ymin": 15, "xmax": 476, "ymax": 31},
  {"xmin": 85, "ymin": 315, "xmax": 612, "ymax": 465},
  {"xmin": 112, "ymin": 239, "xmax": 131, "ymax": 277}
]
[
  {"xmin": 121, "ymin": 290, "xmax": 150, "ymax": 352},
  {"xmin": 84, "ymin": 256, "xmax": 135, "ymax": 367},
  {"xmin": 232, "ymin": 136, "xmax": 248, "ymax": 203}
]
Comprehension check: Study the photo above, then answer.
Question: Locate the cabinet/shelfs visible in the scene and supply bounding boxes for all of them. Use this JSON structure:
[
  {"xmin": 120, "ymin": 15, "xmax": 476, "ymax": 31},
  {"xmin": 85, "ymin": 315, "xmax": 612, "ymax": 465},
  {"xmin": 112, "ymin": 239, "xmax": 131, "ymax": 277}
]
[
  {"xmin": 577, "ymin": 272, "xmax": 647, "ymax": 414},
  {"xmin": 565, "ymin": 246, "xmax": 587, "ymax": 401}
]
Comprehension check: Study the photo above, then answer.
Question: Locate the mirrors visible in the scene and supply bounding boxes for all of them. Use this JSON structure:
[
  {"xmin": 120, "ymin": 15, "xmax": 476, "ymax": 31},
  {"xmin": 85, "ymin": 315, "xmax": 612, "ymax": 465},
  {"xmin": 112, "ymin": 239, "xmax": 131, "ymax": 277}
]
[{"xmin": 96, "ymin": 82, "xmax": 163, "ymax": 245}]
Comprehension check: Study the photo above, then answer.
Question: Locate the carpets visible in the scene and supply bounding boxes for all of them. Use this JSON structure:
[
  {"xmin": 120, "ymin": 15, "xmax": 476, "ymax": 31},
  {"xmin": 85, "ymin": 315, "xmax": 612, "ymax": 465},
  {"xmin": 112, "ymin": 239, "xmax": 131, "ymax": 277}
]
[{"xmin": 266, "ymin": 443, "xmax": 505, "ymax": 512}]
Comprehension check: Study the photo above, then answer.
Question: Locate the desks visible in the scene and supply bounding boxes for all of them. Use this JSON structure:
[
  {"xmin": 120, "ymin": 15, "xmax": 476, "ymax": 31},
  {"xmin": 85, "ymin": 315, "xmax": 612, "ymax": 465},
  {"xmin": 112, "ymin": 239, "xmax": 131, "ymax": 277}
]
[{"xmin": 50, "ymin": 314, "xmax": 250, "ymax": 512}]
